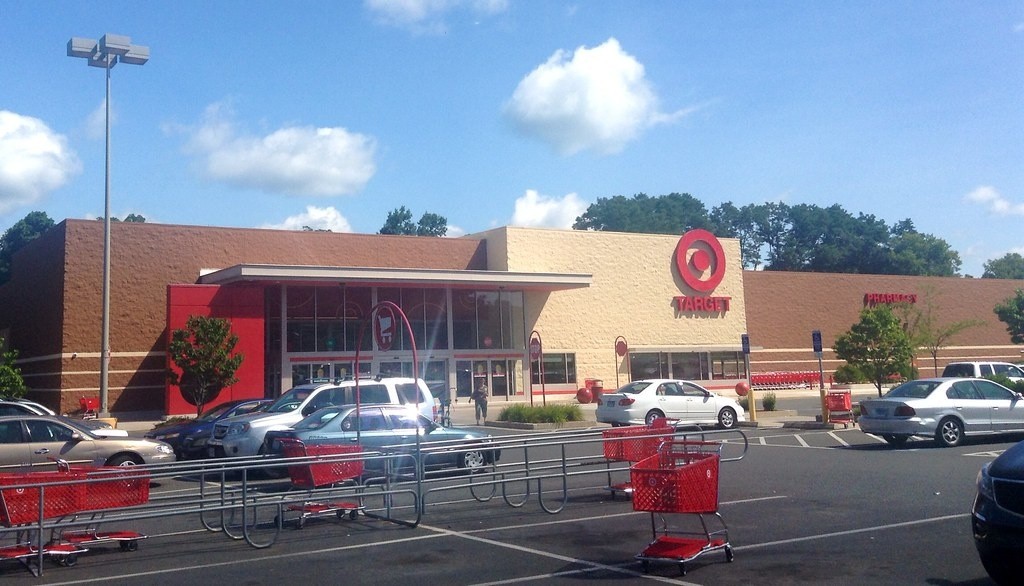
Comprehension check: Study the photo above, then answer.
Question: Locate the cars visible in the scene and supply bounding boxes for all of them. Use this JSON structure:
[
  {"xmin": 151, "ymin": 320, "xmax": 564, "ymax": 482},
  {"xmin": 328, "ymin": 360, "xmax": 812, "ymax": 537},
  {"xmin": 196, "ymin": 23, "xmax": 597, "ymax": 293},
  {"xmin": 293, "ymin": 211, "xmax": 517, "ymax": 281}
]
[
  {"xmin": 595, "ymin": 379, "xmax": 746, "ymax": 429},
  {"xmin": 0, "ymin": 397, "xmax": 176, "ymax": 492},
  {"xmin": 144, "ymin": 398, "xmax": 335, "ymax": 460},
  {"xmin": 970, "ymin": 441, "xmax": 1024, "ymax": 586},
  {"xmin": 858, "ymin": 377, "xmax": 1024, "ymax": 447},
  {"xmin": 260, "ymin": 402, "xmax": 501, "ymax": 488}
]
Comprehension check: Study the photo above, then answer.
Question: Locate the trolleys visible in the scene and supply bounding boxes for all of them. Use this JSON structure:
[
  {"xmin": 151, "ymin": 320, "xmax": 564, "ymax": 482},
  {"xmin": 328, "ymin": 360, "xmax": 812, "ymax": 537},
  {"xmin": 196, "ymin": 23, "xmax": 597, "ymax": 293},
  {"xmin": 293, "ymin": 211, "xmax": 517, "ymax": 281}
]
[
  {"xmin": 0, "ymin": 467, "xmax": 86, "ymax": 567},
  {"xmin": 726, "ymin": 370, "xmax": 820, "ymax": 390},
  {"xmin": 630, "ymin": 440, "xmax": 734, "ymax": 578},
  {"xmin": 79, "ymin": 395, "xmax": 100, "ymax": 419},
  {"xmin": 47, "ymin": 455, "xmax": 151, "ymax": 552},
  {"xmin": 827, "ymin": 385, "xmax": 855, "ymax": 429},
  {"xmin": 274, "ymin": 437, "xmax": 366, "ymax": 530},
  {"xmin": 602, "ymin": 418, "xmax": 682, "ymax": 500}
]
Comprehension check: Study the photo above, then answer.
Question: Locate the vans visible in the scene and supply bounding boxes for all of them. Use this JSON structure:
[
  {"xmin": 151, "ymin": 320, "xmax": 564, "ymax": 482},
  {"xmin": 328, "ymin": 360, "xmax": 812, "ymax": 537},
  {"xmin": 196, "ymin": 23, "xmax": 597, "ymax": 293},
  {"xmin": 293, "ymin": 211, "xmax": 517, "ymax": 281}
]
[{"xmin": 942, "ymin": 361, "xmax": 1024, "ymax": 397}]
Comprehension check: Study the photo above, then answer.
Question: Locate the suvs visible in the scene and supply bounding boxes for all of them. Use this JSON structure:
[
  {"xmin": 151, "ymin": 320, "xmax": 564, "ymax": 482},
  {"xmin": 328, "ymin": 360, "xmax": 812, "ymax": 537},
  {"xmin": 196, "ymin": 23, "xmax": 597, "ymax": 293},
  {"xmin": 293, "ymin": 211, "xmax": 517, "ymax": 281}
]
[{"xmin": 204, "ymin": 376, "xmax": 439, "ymax": 479}]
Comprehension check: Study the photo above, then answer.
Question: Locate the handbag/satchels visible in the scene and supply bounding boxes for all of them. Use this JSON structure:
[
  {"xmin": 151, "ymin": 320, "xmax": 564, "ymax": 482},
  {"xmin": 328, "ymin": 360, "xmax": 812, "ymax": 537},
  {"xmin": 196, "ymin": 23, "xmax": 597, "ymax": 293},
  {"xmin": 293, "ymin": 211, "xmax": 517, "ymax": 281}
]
[{"xmin": 471, "ymin": 390, "xmax": 479, "ymax": 400}]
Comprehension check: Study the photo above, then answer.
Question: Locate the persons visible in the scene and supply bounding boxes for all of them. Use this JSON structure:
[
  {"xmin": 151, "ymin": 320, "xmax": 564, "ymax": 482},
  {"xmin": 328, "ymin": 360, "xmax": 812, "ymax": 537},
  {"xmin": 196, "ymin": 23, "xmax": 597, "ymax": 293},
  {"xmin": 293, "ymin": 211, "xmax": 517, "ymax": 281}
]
[{"xmin": 471, "ymin": 378, "xmax": 488, "ymax": 426}]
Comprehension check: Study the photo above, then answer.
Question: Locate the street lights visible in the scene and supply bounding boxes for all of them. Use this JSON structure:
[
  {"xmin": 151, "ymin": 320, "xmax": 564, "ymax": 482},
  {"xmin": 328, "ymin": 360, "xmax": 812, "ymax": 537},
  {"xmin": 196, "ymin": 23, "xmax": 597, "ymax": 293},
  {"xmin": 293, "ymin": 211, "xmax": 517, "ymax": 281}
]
[{"xmin": 67, "ymin": 33, "xmax": 151, "ymax": 418}]
